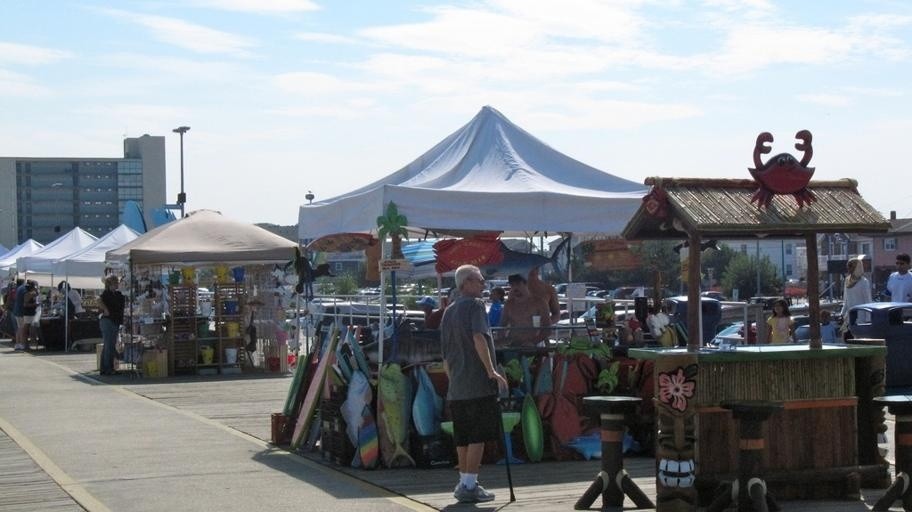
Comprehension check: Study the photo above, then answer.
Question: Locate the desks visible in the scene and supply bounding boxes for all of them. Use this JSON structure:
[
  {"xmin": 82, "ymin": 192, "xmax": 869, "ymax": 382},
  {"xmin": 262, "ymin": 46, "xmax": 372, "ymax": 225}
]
[{"xmin": 627, "ymin": 339, "xmax": 888, "ymax": 504}]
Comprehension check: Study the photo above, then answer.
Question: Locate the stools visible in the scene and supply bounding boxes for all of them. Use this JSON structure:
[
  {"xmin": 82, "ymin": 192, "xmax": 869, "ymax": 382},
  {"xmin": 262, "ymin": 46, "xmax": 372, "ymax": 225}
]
[
  {"xmin": 707, "ymin": 401, "xmax": 784, "ymax": 511},
  {"xmin": 871, "ymin": 396, "xmax": 911, "ymax": 511},
  {"xmin": 575, "ymin": 397, "xmax": 655, "ymax": 512}
]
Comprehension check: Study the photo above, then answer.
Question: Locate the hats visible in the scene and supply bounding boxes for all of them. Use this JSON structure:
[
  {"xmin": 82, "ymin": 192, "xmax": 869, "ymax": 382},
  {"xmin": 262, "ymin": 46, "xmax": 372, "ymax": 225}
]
[
  {"xmin": 416, "ymin": 296, "xmax": 435, "ymax": 306},
  {"xmin": 506, "ymin": 274, "xmax": 527, "ymax": 285}
]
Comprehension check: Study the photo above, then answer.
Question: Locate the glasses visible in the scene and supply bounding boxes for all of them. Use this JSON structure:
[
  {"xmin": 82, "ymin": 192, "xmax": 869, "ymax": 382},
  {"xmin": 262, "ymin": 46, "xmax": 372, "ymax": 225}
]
[{"xmin": 474, "ymin": 278, "xmax": 486, "ymax": 284}]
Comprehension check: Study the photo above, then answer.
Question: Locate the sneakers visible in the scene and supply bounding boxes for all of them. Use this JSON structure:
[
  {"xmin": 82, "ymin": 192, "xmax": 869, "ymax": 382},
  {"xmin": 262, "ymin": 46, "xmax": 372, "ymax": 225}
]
[{"xmin": 453, "ymin": 482, "xmax": 495, "ymax": 501}]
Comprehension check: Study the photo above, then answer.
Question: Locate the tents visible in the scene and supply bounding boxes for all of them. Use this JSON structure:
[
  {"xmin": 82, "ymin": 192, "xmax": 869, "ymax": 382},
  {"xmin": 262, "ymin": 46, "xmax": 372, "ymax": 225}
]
[
  {"xmin": 105, "ymin": 208, "xmax": 309, "ymax": 375},
  {"xmin": 53, "ymin": 223, "xmax": 141, "ymax": 354},
  {"xmin": 392, "ymin": 231, "xmax": 575, "ymax": 329},
  {"xmin": 295, "ymin": 107, "xmax": 653, "ymax": 469},
  {"xmin": 0, "ymin": 239, "xmax": 43, "ymax": 280},
  {"xmin": 16, "ymin": 227, "xmax": 100, "ymax": 351}
]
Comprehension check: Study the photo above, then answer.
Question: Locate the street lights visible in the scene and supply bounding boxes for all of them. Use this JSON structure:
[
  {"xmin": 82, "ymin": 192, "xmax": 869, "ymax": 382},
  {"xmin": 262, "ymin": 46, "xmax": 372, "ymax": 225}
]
[
  {"xmin": 172, "ymin": 125, "xmax": 190, "ymax": 218},
  {"xmin": 51, "ymin": 183, "xmax": 63, "ymax": 238}
]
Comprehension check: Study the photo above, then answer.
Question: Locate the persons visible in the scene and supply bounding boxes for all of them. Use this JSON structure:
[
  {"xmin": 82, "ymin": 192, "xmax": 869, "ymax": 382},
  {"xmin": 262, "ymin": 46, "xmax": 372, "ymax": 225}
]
[
  {"xmin": 840, "ymin": 258, "xmax": 872, "ymax": 344},
  {"xmin": 58, "ymin": 281, "xmax": 75, "ymax": 319},
  {"xmin": 14, "ymin": 279, "xmax": 33, "ymax": 350},
  {"xmin": 766, "ymin": 300, "xmax": 798, "ymax": 344},
  {"xmin": 440, "ymin": 264, "xmax": 508, "ymax": 502},
  {"xmin": 416, "ymin": 296, "xmax": 447, "ymax": 330},
  {"xmin": 820, "ymin": 310, "xmax": 836, "ymax": 344},
  {"xmin": 498, "ymin": 274, "xmax": 552, "ymax": 345},
  {"xmin": 882, "ymin": 253, "xmax": 912, "ymax": 302},
  {"xmin": 96, "ymin": 276, "xmax": 125, "ymax": 375},
  {"xmin": 488, "ymin": 286, "xmax": 505, "ymax": 326},
  {"xmin": 23, "ymin": 286, "xmax": 40, "ymax": 353},
  {"xmin": 508, "ymin": 270, "xmax": 561, "ymax": 324}
]
